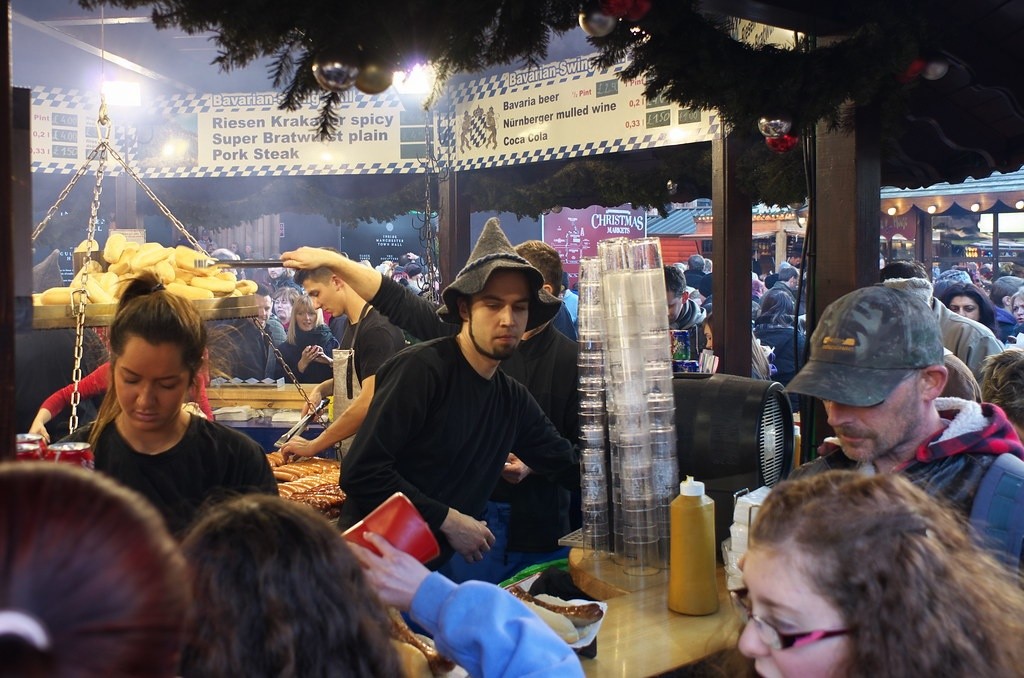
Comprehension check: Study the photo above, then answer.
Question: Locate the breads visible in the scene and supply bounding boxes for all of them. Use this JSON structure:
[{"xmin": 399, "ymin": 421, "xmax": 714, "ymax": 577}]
[{"xmin": 33, "ymin": 233, "xmax": 258, "ymax": 305}]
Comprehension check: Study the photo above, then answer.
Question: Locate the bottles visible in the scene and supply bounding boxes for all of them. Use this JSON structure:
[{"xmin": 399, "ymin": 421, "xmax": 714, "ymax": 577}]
[
  {"xmin": 667, "ymin": 475, "xmax": 720, "ymax": 617},
  {"xmin": 339, "ymin": 492, "xmax": 440, "ymax": 565}
]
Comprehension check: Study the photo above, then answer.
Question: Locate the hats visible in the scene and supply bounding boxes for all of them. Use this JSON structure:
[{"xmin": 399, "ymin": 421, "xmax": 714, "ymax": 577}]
[
  {"xmin": 784, "ymin": 283, "xmax": 944, "ymax": 409},
  {"xmin": 438, "ymin": 219, "xmax": 563, "ymax": 335}
]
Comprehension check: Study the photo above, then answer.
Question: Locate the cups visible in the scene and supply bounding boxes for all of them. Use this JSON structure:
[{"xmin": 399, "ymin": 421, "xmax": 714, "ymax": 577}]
[
  {"xmin": 699, "ymin": 354, "xmax": 719, "ymax": 374},
  {"xmin": 578, "ymin": 236, "xmax": 679, "ymax": 578}
]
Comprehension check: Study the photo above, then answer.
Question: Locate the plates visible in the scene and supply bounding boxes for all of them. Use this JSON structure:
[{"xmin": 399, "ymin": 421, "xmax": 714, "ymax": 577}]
[{"xmin": 532, "ymin": 593, "xmax": 608, "ymax": 649}]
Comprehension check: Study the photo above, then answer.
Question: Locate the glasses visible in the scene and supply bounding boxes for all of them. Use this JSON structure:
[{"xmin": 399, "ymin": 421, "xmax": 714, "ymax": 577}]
[{"xmin": 731, "ymin": 590, "xmax": 851, "ymax": 651}]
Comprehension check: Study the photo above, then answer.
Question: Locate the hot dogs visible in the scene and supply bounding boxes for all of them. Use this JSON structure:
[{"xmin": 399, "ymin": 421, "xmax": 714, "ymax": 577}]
[
  {"xmin": 385, "ymin": 604, "xmax": 458, "ymax": 678},
  {"xmin": 509, "ymin": 585, "xmax": 604, "ymax": 644}
]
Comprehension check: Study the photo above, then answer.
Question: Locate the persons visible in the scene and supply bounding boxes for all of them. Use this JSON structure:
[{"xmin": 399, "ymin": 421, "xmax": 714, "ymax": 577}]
[
  {"xmin": 702, "ymin": 470, "xmax": 1024, "ymax": 678},
  {"xmin": 283, "ymin": 267, "xmax": 406, "ymax": 462},
  {"xmin": 283, "ymin": 293, "xmax": 339, "ymax": 384},
  {"xmin": 933, "ymin": 269, "xmax": 972, "ymax": 284},
  {"xmin": 32, "ymin": 239, "xmax": 1024, "ymax": 381},
  {"xmin": 280, "ymin": 238, "xmax": 580, "ymax": 446},
  {"xmin": 1005, "ymin": 288, "xmax": 1024, "ymax": 348},
  {"xmin": 339, "ymin": 218, "xmax": 581, "ymax": 569},
  {"xmin": 232, "ymin": 286, "xmax": 286, "ymax": 381},
  {"xmin": 980, "ymin": 349, "xmax": 1024, "ymax": 447},
  {"xmin": 991, "ymin": 276, "xmax": 1024, "ymax": 315},
  {"xmin": 0, "ymin": 461, "xmax": 188, "ymax": 678},
  {"xmin": 880, "ymin": 260, "xmax": 1003, "ymax": 392},
  {"xmin": 181, "ymin": 482, "xmax": 582, "ymax": 678},
  {"xmin": 938, "ymin": 282, "xmax": 999, "ymax": 339},
  {"xmin": 784, "ymin": 284, "xmax": 1024, "ymax": 571},
  {"xmin": 46, "ymin": 270, "xmax": 280, "ymax": 537}
]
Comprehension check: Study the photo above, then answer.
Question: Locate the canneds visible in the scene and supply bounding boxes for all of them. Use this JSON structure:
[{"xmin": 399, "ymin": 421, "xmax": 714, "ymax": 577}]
[
  {"xmin": 16, "ymin": 433, "xmax": 95, "ymax": 471},
  {"xmin": 668, "ymin": 330, "xmax": 700, "ymax": 372}
]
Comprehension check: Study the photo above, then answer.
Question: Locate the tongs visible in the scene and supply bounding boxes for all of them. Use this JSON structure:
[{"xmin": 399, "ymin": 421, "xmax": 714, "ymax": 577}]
[
  {"xmin": 193, "ymin": 257, "xmax": 283, "ymax": 267},
  {"xmin": 273, "ymin": 397, "xmax": 330, "ymax": 448}
]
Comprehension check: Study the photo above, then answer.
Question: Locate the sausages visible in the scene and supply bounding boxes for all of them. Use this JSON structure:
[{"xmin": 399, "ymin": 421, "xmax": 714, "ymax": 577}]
[{"xmin": 264, "ymin": 451, "xmax": 347, "ymax": 511}]
